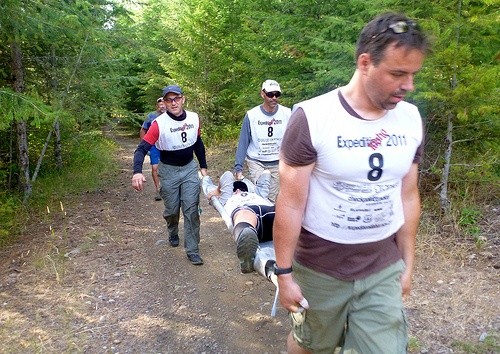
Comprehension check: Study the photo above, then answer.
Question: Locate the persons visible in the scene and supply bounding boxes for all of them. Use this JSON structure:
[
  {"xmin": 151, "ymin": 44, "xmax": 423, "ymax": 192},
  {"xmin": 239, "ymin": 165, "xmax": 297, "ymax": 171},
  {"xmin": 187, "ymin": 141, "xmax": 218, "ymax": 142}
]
[
  {"xmin": 207, "ymin": 169, "xmax": 276, "ymax": 274},
  {"xmin": 273, "ymin": 14, "xmax": 426, "ymax": 354},
  {"xmin": 234, "ymin": 80, "xmax": 292, "ymax": 206},
  {"xmin": 131, "ymin": 85, "xmax": 208, "ymax": 265},
  {"xmin": 141, "ymin": 98, "xmax": 167, "ymax": 201}
]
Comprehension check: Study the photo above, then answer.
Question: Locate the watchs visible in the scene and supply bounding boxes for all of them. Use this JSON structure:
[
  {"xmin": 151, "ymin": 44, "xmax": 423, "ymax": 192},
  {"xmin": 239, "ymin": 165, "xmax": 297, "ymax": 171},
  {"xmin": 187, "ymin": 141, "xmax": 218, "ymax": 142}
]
[{"xmin": 274, "ymin": 264, "xmax": 294, "ymax": 275}]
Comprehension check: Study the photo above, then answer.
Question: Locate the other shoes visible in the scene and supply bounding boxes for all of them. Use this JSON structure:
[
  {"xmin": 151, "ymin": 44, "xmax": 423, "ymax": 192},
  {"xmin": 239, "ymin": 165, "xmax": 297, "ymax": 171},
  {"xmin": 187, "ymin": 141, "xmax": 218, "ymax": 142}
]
[
  {"xmin": 169, "ymin": 233, "xmax": 179, "ymax": 247},
  {"xmin": 236, "ymin": 227, "xmax": 259, "ymax": 274},
  {"xmin": 155, "ymin": 191, "xmax": 161, "ymax": 201},
  {"xmin": 186, "ymin": 254, "xmax": 204, "ymax": 265}
]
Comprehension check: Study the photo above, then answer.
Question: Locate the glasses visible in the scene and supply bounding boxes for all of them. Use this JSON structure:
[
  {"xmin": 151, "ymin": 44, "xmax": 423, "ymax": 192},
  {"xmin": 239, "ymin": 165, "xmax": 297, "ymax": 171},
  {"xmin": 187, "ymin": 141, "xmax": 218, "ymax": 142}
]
[
  {"xmin": 163, "ymin": 97, "xmax": 183, "ymax": 103},
  {"xmin": 362, "ymin": 21, "xmax": 422, "ymax": 50},
  {"xmin": 263, "ymin": 90, "xmax": 282, "ymax": 98}
]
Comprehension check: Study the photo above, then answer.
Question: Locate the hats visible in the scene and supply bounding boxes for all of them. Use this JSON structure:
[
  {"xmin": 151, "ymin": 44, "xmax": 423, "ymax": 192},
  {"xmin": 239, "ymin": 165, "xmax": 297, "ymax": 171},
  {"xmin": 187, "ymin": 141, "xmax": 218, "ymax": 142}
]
[
  {"xmin": 262, "ymin": 79, "xmax": 281, "ymax": 92},
  {"xmin": 163, "ymin": 85, "xmax": 183, "ymax": 99},
  {"xmin": 157, "ymin": 97, "xmax": 164, "ymax": 103},
  {"xmin": 232, "ymin": 180, "xmax": 249, "ymax": 193}
]
